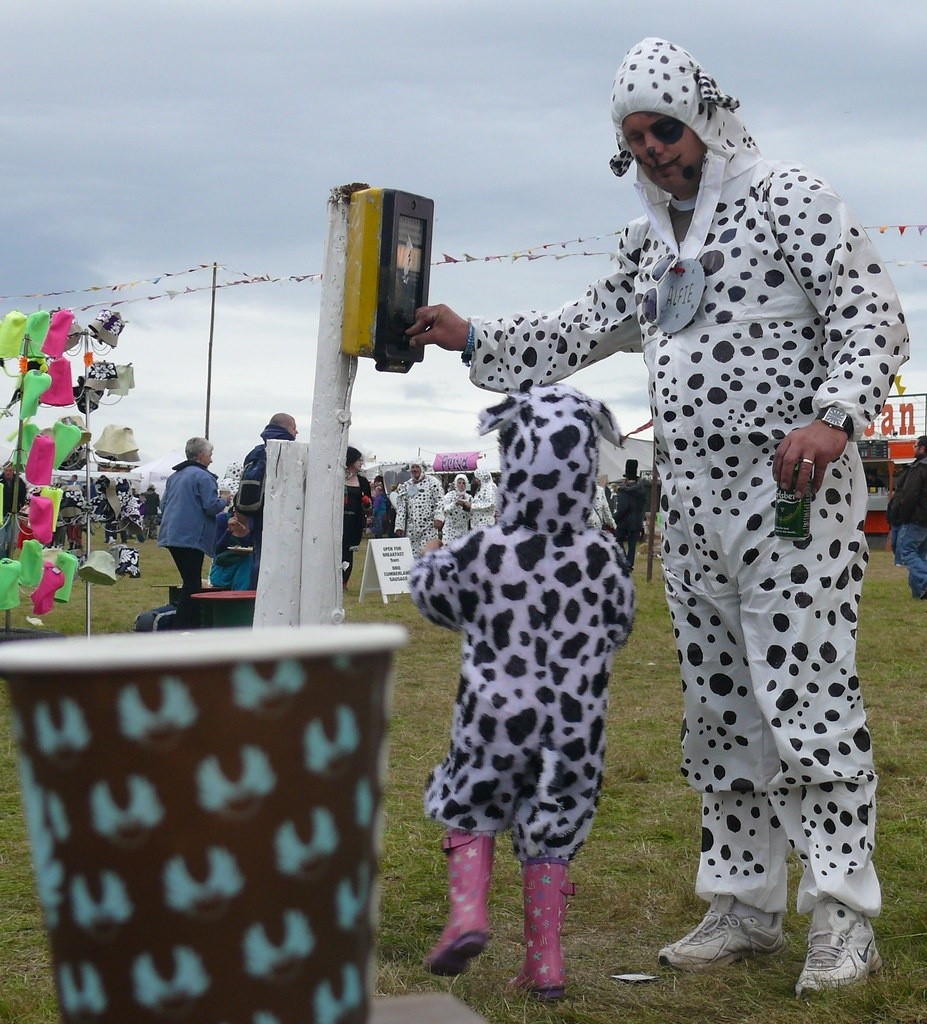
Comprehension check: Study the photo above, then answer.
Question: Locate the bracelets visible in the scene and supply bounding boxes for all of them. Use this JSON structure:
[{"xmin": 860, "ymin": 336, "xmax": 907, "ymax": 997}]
[{"xmin": 462, "ymin": 320, "xmax": 475, "ymax": 366}]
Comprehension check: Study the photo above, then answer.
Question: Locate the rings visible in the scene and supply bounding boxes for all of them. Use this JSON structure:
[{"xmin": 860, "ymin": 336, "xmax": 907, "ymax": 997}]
[{"xmin": 801, "ymin": 458, "xmax": 814, "ymax": 465}]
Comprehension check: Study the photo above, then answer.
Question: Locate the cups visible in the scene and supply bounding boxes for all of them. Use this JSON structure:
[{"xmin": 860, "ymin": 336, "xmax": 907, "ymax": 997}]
[
  {"xmin": 220, "ymin": 490, "xmax": 230, "ymax": 506},
  {"xmin": 0, "ymin": 623, "xmax": 424, "ymax": 1024}
]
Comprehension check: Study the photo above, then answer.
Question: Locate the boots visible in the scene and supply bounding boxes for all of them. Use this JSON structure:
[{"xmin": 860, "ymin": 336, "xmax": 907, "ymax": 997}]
[
  {"xmin": 424, "ymin": 829, "xmax": 495, "ymax": 977},
  {"xmin": 504, "ymin": 856, "xmax": 577, "ymax": 999}
]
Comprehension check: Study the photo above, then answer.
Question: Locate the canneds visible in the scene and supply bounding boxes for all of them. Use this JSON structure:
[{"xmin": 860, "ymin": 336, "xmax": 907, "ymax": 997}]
[{"xmin": 774, "ymin": 458, "xmax": 813, "ymax": 541}]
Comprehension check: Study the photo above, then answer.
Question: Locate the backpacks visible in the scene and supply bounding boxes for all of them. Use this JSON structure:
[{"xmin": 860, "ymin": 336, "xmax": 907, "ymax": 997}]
[{"xmin": 234, "ymin": 444, "xmax": 268, "ymax": 517}]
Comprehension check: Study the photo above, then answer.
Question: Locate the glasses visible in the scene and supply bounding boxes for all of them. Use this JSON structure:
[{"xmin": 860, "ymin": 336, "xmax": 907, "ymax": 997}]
[{"xmin": 641, "ymin": 252, "xmax": 679, "ymax": 325}]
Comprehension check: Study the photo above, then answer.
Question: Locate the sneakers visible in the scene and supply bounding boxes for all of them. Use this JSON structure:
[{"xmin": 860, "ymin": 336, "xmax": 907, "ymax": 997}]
[
  {"xmin": 657, "ymin": 893, "xmax": 783, "ymax": 971},
  {"xmin": 795, "ymin": 893, "xmax": 882, "ymax": 998}
]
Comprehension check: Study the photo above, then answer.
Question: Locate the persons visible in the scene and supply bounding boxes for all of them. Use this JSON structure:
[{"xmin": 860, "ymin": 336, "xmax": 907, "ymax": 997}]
[
  {"xmin": 406, "ymin": 383, "xmax": 636, "ymax": 999},
  {"xmin": 406, "ymin": 38, "xmax": 912, "ymax": 999},
  {"xmin": 0, "ymin": 412, "xmax": 504, "ymax": 593},
  {"xmin": 885, "ymin": 436, "xmax": 927, "ymax": 602},
  {"xmin": 586, "ymin": 459, "xmax": 646, "ymax": 578}
]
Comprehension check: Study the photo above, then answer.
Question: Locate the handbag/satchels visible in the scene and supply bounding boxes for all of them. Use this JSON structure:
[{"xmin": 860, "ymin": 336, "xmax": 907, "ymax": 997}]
[{"xmin": 601, "ymin": 523, "xmax": 614, "ymax": 534}]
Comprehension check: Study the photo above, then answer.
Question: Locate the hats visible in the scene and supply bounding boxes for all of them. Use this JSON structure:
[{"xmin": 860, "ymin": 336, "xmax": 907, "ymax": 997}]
[
  {"xmin": 622, "ymin": 459, "xmax": 640, "ymax": 478},
  {"xmin": 0, "ymin": 308, "xmax": 142, "ymax": 616},
  {"xmin": 391, "ymin": 485, "xmax": 397, "ymax": 491},
  {"xmin": 147, "ymin": 484, "xmax": 156, "ymax": 489}
]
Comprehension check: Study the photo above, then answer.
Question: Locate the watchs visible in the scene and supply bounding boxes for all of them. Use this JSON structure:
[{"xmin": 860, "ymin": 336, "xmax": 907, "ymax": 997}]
[{"xmin": 814, "ymin": 405, "xmax": 854, "ymax": 440}]
